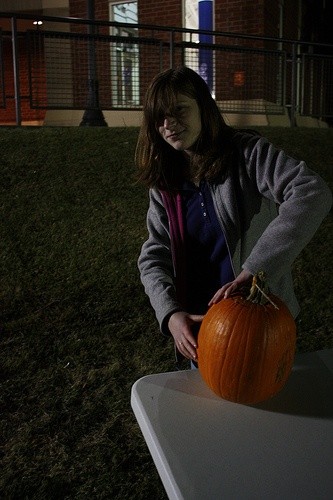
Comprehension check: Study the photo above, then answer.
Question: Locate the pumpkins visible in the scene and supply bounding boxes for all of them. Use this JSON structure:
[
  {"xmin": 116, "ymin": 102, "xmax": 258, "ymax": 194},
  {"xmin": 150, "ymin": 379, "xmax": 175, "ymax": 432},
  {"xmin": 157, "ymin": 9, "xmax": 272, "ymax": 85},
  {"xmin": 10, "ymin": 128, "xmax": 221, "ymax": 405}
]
[{"xmin": 197, "ymin": 271, "xmax": 296, "ymax": 405}]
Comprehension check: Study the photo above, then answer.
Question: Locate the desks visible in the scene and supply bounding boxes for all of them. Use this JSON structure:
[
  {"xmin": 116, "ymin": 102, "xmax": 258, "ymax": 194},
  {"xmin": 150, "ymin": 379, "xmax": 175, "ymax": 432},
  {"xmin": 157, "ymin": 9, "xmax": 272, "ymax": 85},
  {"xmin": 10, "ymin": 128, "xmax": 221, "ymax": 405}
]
[{"xmin": 132, "ymin": 349, "xmax": 332, "ymax": 499}]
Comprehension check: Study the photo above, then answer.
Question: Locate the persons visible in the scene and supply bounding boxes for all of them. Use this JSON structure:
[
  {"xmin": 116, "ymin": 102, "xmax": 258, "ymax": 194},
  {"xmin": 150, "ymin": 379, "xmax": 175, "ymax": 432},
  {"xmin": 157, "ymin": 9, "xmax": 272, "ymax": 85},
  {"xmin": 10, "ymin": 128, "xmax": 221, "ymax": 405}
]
[{"xmin": 128, "ymin": 68, "xmax": 333, "ymax": 371}]
